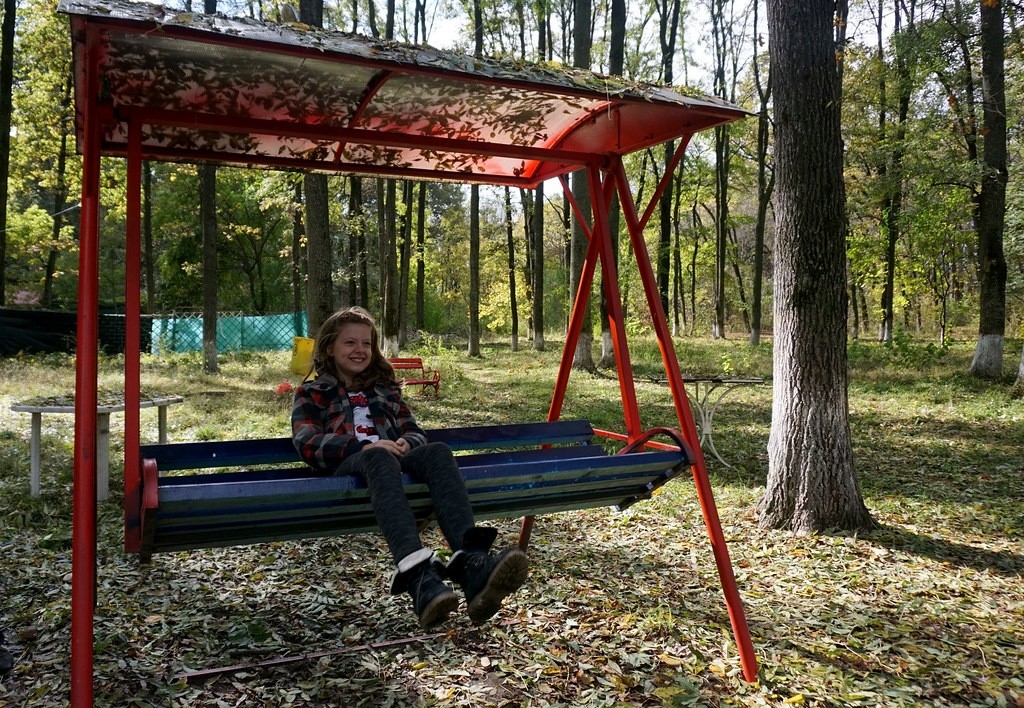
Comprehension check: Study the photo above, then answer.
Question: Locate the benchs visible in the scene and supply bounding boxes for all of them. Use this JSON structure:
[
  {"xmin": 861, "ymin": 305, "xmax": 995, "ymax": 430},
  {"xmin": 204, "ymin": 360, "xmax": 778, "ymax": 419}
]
[{"xmin": 386, "ymin": 358, "xmax": 441, "ymax": 399}]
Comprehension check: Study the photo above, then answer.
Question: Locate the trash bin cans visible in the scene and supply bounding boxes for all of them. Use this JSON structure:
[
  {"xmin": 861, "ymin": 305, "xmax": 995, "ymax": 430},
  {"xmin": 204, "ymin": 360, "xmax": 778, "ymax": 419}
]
[{"xmin": 289, "ymin": 336, "xmax": 314, "ymax": 376}]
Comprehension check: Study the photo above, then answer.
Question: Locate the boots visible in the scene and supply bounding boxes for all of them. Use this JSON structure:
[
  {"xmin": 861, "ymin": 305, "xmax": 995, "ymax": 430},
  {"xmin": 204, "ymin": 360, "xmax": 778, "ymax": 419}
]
[
  {"xmin": 389, "ymin": 545, "xmax": 460, "ymax": 630},
  {"xmin": 445, "ymin": 527, "xmax": 528, "ymax": 624}
]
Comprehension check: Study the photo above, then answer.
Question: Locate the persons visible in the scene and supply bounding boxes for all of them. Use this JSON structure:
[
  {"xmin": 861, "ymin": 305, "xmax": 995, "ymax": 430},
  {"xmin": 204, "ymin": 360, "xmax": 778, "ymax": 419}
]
[{"xmin": 291, "ymin": 305, "xmax": 529, "ymax": 625}]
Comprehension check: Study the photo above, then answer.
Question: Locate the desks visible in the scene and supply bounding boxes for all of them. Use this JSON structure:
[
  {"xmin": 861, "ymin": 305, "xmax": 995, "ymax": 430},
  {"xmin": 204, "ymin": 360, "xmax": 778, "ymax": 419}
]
[
  {"xmin": 11, "ymin": 393, "xmax": 183, "ymax": 502},
  {"xmin": 651, "ymin": 378, "xmax": 764, "ymax": 468}
]
